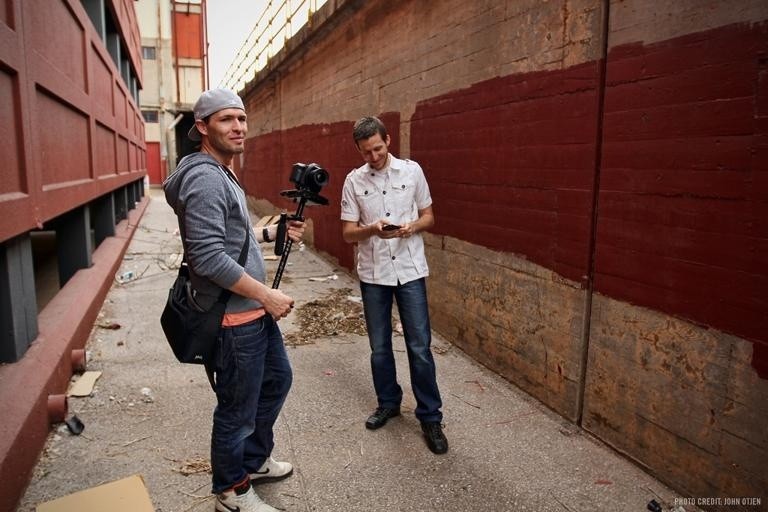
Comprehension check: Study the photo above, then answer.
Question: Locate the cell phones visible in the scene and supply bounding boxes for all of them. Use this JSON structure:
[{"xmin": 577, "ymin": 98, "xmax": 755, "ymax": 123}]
[{"xmin": 382, "ymin": 224, "xmax": 401, "ymax": 231}]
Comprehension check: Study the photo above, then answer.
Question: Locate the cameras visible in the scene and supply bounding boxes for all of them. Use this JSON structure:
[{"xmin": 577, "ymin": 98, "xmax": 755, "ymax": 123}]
[{"xmin": 288, "ymin": 162, "xmax": 330, "ymax": 195}]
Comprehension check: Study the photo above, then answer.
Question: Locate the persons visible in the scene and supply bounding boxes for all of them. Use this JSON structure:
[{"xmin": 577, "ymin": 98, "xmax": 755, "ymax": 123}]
[
  {"xmin": 338, "ymin": 114, "xmax": 450, "ymax": 455},
  {"xmin": 160, "ymin": 87, "xmax": 308, "ymax": 511}
]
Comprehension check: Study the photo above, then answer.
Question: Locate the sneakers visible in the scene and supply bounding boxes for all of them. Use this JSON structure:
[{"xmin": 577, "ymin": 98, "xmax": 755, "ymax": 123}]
[
  {"xmin": 365, "ymin": 405, "xmax": 400, "ymax": 429},
  {"xmin": 214, "ymin": 475, "xmax": 279, "ymax": 512},
  {"xmin": 249, "ymin": 458, "xmax": 292, "ymax": 486},
  {"xmin": 420, "ymin": 421, "xmax": 448, "ymax": 453}
]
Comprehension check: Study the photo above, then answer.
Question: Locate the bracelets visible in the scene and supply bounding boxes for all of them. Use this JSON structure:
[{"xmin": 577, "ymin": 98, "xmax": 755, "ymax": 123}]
[{"xmin": 261, "ymin": 226, "xmax": 276, "ymax": 244}]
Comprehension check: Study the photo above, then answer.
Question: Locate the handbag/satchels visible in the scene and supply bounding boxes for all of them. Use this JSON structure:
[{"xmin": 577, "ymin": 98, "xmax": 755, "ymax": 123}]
[{"xmin": 160, "ymin": 266, "xmax": 231, "ymax": 363}]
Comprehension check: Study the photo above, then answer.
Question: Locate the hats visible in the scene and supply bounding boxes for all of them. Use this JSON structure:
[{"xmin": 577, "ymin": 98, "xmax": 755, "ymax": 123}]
[{"xmin": 187, "ymin": 87, "xmax": 245, "ymax": 141}]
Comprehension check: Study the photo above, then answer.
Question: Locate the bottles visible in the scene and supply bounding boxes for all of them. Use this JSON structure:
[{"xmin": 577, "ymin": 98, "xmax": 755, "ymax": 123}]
[{"xmin": 120, "ymin": 271, "xmax": 133, "ymax": 280}]
